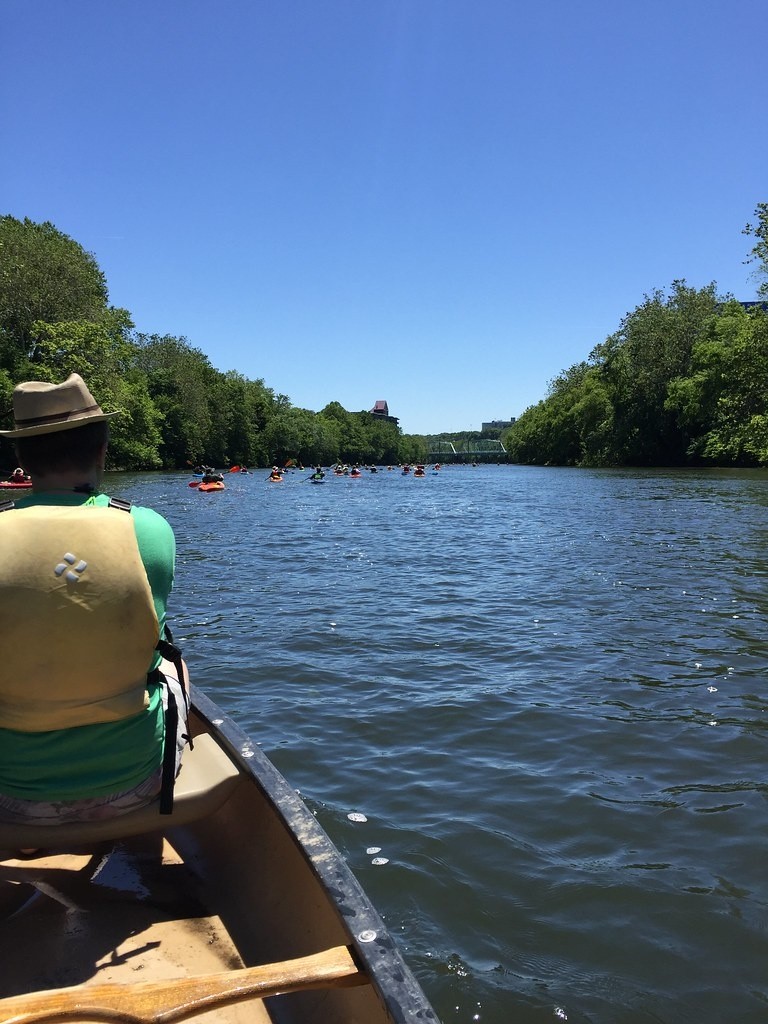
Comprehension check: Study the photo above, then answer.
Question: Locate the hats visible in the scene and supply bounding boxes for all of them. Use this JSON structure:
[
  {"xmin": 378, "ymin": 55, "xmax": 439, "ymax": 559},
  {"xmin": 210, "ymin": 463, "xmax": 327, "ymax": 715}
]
[{"xmin": 0, "ymin": 373, "xmax": 122, "ymax": 438}]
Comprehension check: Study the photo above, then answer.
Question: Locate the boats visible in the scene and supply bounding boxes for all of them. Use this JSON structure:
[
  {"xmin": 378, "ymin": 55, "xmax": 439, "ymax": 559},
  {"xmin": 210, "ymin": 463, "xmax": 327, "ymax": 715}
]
[
  {"xmin": 193, "ymin": 473, "xmax": 206, "ymax": 477},
  {"xmin": 312, "ymin": 479, "xmax": 326, "ymax": 483},
  {"xmin": 281, "ymin": 469, "xmax": 291, "ymax": 473},
  {"xmin": 0, "ymin": 480, "xmax": 33, "ymax": 487},
  {"xmin": 269, "ymin": 476, "xmax": 283, "ymax": 482},
  {"xmin": 333, "ymin": 462, "xmax": 510, "ymax": 477},
  {"xmin": 198, "ymin": 481, "xmax": 225, "ymax": 491},
  {"xmin": 241, "ymin": 472, "xmax": 249, "ymax": 474},
  {"xmin": 298, "ymin": 469, "xmax": 306, "ymax": 472},
  {"xmin": 309, "ymin": 467, "xmax": 316, "ymax": 471}
]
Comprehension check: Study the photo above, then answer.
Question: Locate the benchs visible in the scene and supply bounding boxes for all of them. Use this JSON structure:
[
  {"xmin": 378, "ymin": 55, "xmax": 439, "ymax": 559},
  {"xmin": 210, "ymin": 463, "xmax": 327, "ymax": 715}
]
[{"xmin": 0, "ymin": 731, "xmax": 240, "ymax": 875}]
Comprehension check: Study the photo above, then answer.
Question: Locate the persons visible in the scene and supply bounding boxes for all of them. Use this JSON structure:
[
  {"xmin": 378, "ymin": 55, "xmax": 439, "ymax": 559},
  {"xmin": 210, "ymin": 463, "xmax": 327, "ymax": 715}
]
[
  {"xmin": 0, "ymin": 374, "xmax": 190, "ymax": 861},
  {"xmin": 193, "ymin": 463, "xmax": 509, "ymax": 483},
  {"xmin": 202, "ymin": 468, "xmax": 224, "ymax": 483},
  {"xmin": 8, "ymin": 468, "xmax": 31, "ymax": 484}
]
